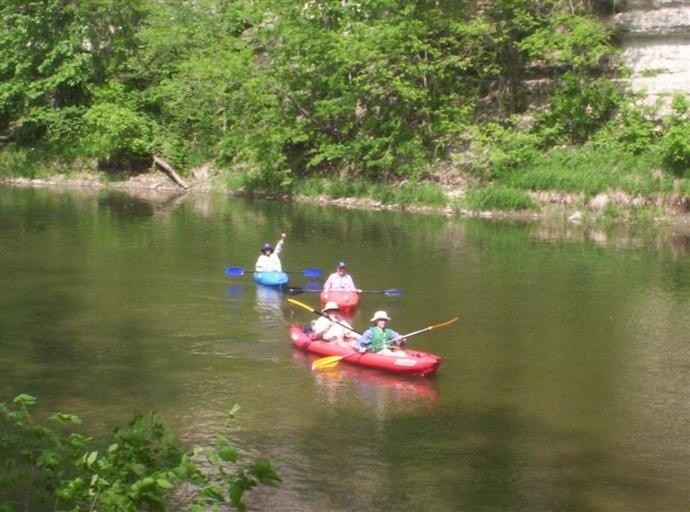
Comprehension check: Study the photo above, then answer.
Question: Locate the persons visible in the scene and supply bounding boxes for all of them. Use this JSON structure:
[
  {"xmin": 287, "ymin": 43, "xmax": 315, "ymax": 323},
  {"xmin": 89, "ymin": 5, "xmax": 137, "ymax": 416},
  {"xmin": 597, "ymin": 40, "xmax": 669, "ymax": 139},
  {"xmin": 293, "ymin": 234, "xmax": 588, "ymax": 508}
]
[
  {"xmin": 312, "ymin": 302, "xmax": 359, "ymax": 343},
  {"xmin": 255, "ymin": 232, "xmax": 287, "ymax": 273},
  {"xmin": 352, "ymin": 310, "xmax": 406, "ymax": 353},
  {"xmin": 322, "ymin": 261, "xmax": 361, "ymax": 299}
]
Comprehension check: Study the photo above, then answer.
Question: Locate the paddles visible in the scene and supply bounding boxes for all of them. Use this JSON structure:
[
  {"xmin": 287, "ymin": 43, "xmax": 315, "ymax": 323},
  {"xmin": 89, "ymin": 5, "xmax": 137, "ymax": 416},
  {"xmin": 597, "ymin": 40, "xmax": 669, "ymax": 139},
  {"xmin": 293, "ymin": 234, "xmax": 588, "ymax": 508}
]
[
  {"xmin": 313, "ymin": 317, "xmax": 459, "ymax": 369},
  {"xmin": 224, "ymin": 266, "xmax": 321, "ymax": 279},
  {"xmin": 287, "ymin": 298, "xmax": 363, "ymax": 337},
  {"xmin": 283, "ymin": 285, "xmax": 406, "ymax": 297}
]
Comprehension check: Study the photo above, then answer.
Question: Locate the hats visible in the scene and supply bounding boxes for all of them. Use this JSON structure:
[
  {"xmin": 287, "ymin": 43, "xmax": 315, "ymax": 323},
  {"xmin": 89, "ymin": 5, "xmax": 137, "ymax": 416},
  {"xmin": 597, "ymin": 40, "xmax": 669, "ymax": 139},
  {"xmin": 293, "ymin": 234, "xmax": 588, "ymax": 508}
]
[
  {"xmin": 321, "ymin": 301, "xmax": 340, "ymax": 312},
  {"xmin": 261, "ymin": 243, "xmax": 274, "ymax": 253},
  {"xmin": 370, "ymin": 311, "xmax": 391, "ymax": 322},
  {"xmin": 338, "ymin": 262, "xmax": 346, "ymax": 269}
]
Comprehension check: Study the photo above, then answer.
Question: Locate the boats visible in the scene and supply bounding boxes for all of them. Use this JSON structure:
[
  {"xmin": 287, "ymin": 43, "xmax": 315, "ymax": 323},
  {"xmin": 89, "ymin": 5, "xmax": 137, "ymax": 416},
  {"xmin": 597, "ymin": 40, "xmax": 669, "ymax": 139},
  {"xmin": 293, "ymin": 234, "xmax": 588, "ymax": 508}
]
[
  {"xmin": 251, "ymin": 270, "xmax": 289, "ymax": 286},
  {"xmin": 289, "ymin": 323, "xmax": 442, "ymax": 376},
  {"xmin": 292, "ymin": 347, "xmax": 440, "ymax": 404},
  {"xmin": 255, "ymin": 284, "xmax": 282, "ymax": 312}
]
